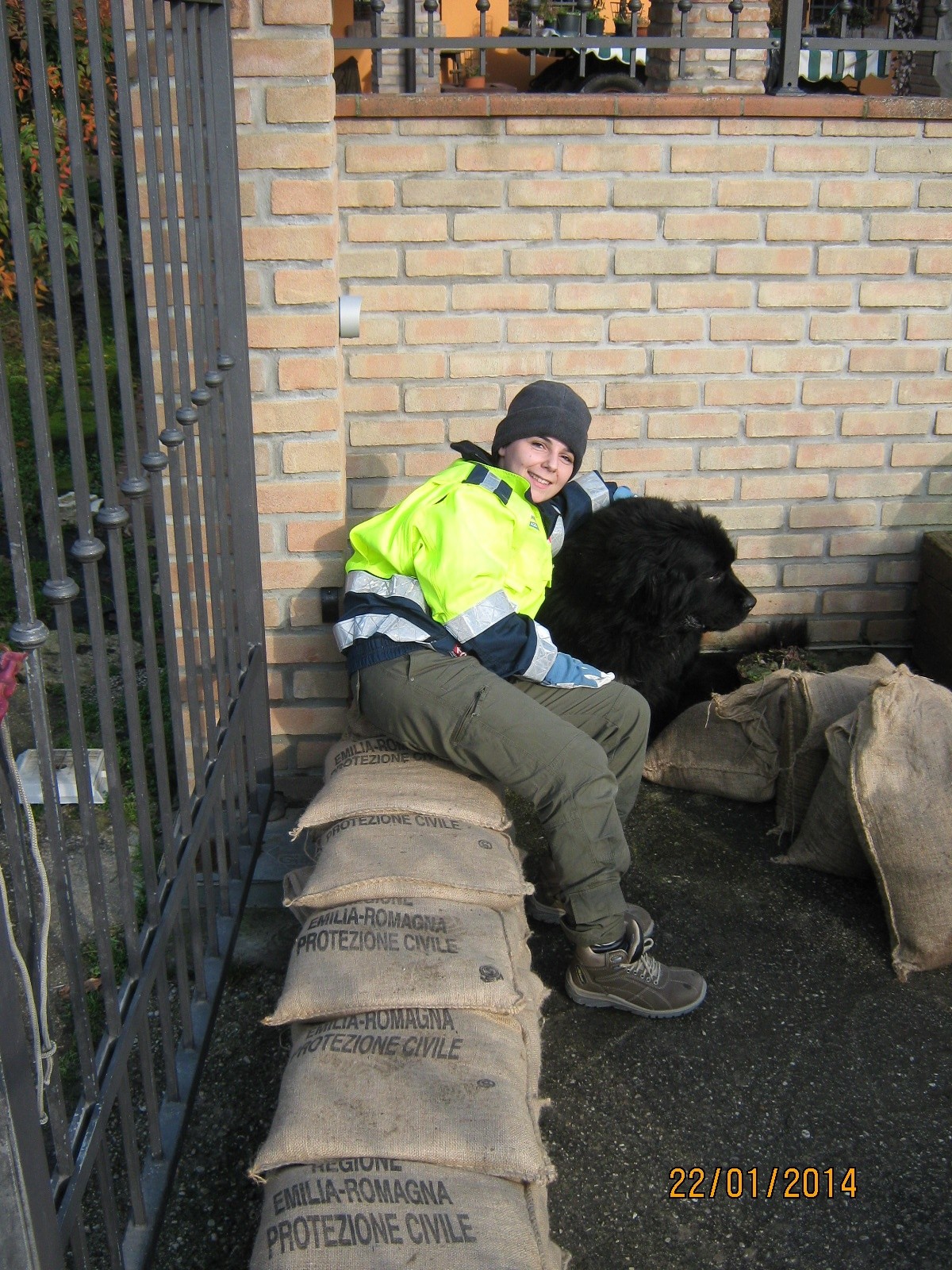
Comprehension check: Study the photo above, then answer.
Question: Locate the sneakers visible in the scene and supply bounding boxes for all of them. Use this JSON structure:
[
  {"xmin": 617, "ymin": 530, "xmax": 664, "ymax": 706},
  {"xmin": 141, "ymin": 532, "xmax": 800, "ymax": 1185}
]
[
  {"xmin": 565, "ymin": 917, "xmax": 707, "ymax": 1019},
  {"xmin": 529, "ymin": 882, "xmax": 654, "ymax": 940}
]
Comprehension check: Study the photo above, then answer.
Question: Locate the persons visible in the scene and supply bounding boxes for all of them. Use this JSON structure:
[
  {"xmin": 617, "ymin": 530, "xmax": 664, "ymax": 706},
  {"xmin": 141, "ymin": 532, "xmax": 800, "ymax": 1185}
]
[{"xmin": 332, "ymin": 382, "xmax": 708, "ymax": 1020}]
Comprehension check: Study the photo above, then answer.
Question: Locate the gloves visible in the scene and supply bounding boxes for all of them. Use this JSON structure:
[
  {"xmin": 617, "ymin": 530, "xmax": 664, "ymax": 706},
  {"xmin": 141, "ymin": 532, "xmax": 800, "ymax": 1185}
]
[{"xmin": 538, "ymin": 652, "xmax": 615, "ymax": 690}]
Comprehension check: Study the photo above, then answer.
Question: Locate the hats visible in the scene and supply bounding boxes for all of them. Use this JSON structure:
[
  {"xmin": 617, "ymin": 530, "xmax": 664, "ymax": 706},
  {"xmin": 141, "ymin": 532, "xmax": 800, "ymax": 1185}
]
[{"xmin": 492, "ymin": 380, "xmax": 593, "ymax": 482}]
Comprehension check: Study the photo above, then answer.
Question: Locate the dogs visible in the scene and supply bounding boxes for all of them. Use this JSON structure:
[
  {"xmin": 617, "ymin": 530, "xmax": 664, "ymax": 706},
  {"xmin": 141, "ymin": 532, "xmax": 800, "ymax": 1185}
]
[{"xmin": 535, "ymin": 496, "xmax": 810, "ymax": 726}]
